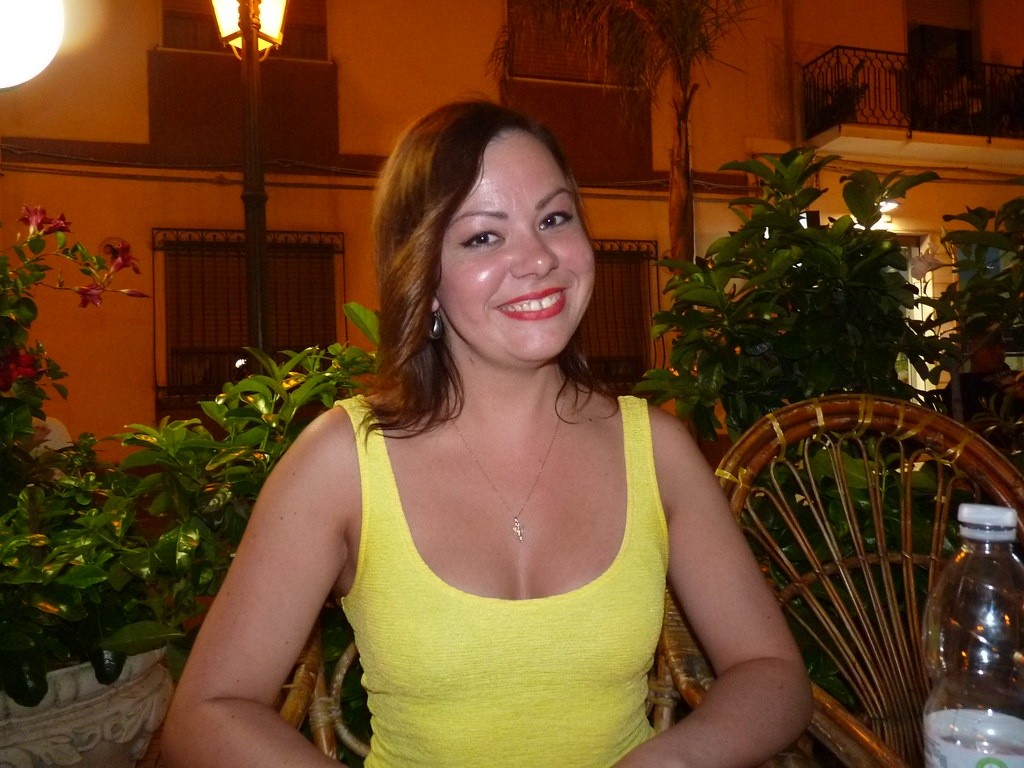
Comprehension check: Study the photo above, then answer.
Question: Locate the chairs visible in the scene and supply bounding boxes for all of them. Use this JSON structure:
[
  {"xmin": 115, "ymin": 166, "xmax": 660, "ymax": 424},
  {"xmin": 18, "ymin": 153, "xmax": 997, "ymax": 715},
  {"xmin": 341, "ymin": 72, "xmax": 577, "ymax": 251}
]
[{"xmin": 277, "ymin": 392, "xmax": 1024, "ymax": 768}]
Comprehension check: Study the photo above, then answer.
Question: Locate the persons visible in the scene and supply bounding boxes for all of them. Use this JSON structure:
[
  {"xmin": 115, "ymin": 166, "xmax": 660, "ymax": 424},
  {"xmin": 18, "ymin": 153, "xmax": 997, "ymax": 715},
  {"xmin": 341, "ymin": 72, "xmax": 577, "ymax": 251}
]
[{"xmin": 160, "ymin": 101, "xmax": 816, "ymax": 768}]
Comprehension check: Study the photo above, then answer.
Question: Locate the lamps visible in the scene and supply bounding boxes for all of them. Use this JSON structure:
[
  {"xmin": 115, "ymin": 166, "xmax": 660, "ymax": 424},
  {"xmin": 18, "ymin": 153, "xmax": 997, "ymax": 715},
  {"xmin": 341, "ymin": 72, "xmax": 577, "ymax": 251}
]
[{"xmin": 875, "ymin": 199, "xmax": 902, "ymax": 212}]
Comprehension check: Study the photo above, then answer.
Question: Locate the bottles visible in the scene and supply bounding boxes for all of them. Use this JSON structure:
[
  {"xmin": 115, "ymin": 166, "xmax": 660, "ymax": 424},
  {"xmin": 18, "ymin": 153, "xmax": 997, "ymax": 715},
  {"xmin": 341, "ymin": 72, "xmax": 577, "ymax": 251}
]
[{"xmin": 922, "ymin": 503, "xmax": 1024, "ymax": 768}]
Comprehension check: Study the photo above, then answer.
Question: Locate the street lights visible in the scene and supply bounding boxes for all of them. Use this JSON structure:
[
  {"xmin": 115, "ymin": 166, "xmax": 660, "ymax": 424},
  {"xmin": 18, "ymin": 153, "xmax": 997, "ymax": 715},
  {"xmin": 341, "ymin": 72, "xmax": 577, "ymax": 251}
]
[{"xmin": 209, "ymin": 0, "xmax": 289, "ymax": 376}]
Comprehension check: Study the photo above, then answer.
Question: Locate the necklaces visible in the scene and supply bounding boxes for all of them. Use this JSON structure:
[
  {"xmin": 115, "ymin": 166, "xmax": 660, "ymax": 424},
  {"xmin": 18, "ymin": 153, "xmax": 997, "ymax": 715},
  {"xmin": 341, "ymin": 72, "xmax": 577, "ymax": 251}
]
[{"xmin": 443, "ymin": 359, "xmax": 567, "ymax": 544}]
